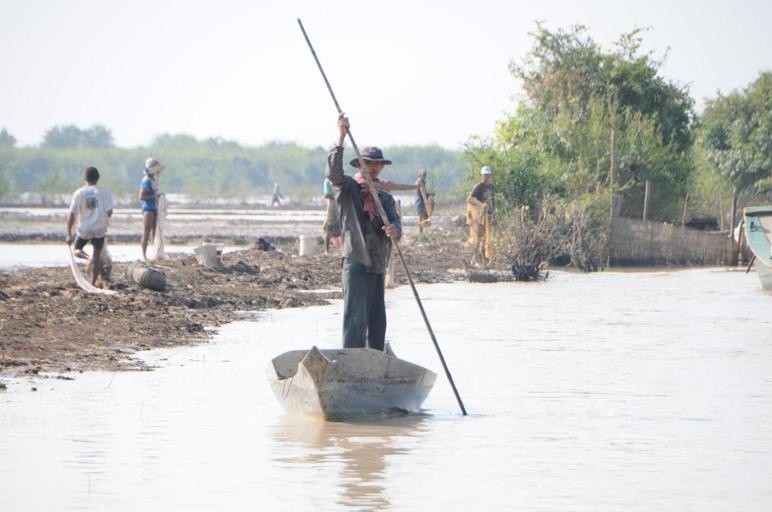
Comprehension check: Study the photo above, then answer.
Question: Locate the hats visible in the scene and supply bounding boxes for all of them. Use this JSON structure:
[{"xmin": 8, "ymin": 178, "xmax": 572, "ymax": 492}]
[
  {"xmin": 349, "ymin": 145, "xmax": 392, "ymax": 169},
  {"xmin": 143, "ymin": 158, "xmax": 165, "ymax": 176},
  {"xmin": 480, "ymin": 166, "xmax": 493, "ymax": 175},
  {"xmin": 417, "ymin": 168, "xmax": 427, "ymax": 177}
]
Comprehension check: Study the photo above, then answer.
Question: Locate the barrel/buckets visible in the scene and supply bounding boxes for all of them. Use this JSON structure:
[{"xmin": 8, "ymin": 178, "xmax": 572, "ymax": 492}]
[
  {"xmin": 204, "ymin": 242, "xmax": 223, "ymax": 265},
  {"xmin": 299, "ymin": 235, "xmax": 317, "ymax": 256},
  {"xmin": 193, "ymin": 245, "xmax": 217, "ymax": 268}
]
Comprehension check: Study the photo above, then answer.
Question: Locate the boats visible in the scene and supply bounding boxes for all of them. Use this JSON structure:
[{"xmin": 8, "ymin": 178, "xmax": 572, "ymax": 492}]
[
  {"xmin": 744, "ymin": 206, "xmax": 772, "ymax": 289},
  {"xmin": 271, "ymin": 346, "xmax": 437, "ymax": 418}
]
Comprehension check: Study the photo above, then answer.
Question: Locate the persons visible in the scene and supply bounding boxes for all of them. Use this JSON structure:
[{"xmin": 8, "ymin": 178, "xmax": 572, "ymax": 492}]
[
  {"xmin": 327, "ymin": 110, "xmax": 403, "ymax": 353},
  {"xmin": 139, "ymin": 158, "xmax": 164, "ymax": 260},
  {"xmin": 466, "ymin": 166, "xmax": 497, "ymax": 271},
  {"xmin": 65, "ymin": 166, "xmax": 113, "ymax": 286},
  {"xmin": 415, "ymin": 169, "xmax": 435, "ymax": 234},
  {"xmin": 271, "ymin": 182, "xmax": 284, "ymax": 208},
  {"xmin": 323, "ymin": 177, "xmax": 342, "ymax": 256}
]
